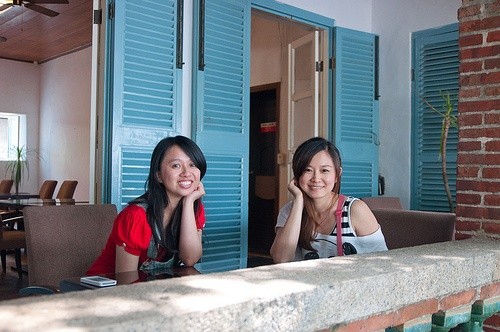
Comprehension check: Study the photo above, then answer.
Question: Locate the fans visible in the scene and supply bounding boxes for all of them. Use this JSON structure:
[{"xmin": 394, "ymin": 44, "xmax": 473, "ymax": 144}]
[{"xmin": 0, "ymin": 0, "xmax": 69, "ymax": 18}]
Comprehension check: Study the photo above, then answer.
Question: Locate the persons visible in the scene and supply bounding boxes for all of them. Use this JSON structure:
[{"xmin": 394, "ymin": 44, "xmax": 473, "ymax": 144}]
[
  {"xmin": 85, "ymin": 136, "xmax": 205, "ymax": 280},
  {"xmin": 270, "ymin": 137, "xmax": 388, "ymax": 265},
  {"xmin": 112, "ymin": 266, "xmax": 201, "ymax": 284}
]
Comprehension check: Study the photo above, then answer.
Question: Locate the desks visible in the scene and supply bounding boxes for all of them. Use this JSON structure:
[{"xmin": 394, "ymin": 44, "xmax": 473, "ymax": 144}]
[
  {"xmin": 0, "ymin": 198, "xmax": 91, "ymax": 207},
  {"xmin": 60, "ymin": 255, "xmax": 275, "ymax": 290}
]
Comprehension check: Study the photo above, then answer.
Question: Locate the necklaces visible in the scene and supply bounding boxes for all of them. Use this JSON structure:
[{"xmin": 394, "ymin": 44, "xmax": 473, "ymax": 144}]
[{"xmin": 314, "ymin": 196, "xmax": 338, "ymax": 228}]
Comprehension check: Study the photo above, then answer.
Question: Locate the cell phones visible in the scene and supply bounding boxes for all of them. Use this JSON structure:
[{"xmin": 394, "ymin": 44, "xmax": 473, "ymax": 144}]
[{"xmin": 81, "ymin": 275, "xmax": 117, "ymax": 287}]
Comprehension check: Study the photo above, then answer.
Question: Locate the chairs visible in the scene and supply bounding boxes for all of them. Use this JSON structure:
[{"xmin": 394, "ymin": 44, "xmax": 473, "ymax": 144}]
[
  {"xmin": 0, "ymin": 213, "xmax": 26, "ymax": 279},
  {"xmin": 361, "ymin": 197, "xmax": 402, "ymax": 210},
  {"xmin": 39, "ymin": 181, "xmax": 58, "ymax": 199},
  {"xmin": 372, "ymin": 209, "xmax": 457, "ymax": 250},
  {"xmin": 18, "ymin": 204, "xmax": 118, "ymax": 295},
  {"xmin": 0, "ymin": 180, "xmax": 14, "ymax": 195},
  {"xmin": 56, "ymin": 180, "xmax": 78, "ymax": 198}
]
